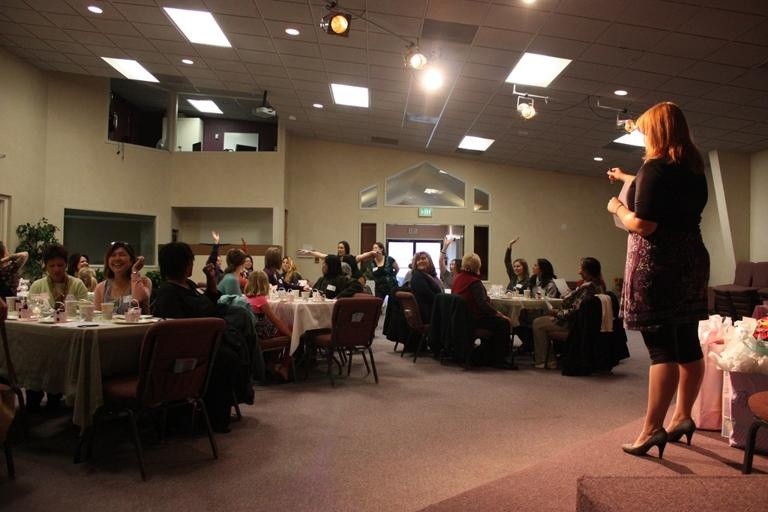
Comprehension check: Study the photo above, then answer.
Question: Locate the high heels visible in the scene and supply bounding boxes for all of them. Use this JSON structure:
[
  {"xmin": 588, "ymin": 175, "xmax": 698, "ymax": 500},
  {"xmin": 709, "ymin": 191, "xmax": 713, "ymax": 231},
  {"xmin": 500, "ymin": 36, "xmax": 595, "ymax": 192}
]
[
  {"xmin": 667, "ymin": 419, "xmax": 696, "ymax": 446},
  {"xmin": 622, "ymin": 428, "xmax": 668, "ymax": 459}
]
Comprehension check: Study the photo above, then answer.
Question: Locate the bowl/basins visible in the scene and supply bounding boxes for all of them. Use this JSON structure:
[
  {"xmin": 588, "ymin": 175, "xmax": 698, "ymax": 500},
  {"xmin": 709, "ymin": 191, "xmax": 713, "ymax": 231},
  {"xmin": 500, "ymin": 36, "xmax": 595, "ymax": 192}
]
[{"xmin": 490, "ymin": 288, "xmax": 503, "ymax": 297}]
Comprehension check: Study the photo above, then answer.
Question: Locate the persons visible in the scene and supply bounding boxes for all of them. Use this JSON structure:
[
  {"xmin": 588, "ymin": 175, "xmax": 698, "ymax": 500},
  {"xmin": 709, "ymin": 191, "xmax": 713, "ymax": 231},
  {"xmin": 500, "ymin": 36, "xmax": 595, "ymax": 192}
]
[
  {"xmin": 504, "ymin": 237, "xmax": 606, "ymax": 369},
  {"xmin": 607, "ymin": 101, "xmax": 711, "ymax": 458},
  {"xmin": 1, "ymin": 240, "xmax": 218, "ymax": 409},
  {"xmin": 207, "ymin": 231, "xmax": 382, "ymax": 369},
  {"xmin": 406, "ymin": 236, "xmax": 519, "ymax": 371},
  {"xmin": 367, "ymin": 242, "xmax": 400, "ymax": 302}
]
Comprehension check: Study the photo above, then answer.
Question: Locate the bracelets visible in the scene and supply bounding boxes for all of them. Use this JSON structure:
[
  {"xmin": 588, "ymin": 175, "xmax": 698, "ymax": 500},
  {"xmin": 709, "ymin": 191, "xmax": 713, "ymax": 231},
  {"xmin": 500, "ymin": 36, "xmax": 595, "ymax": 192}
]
[{"xmin": 615, "ymin": 204, "xmax": 623, "ymax": 215}]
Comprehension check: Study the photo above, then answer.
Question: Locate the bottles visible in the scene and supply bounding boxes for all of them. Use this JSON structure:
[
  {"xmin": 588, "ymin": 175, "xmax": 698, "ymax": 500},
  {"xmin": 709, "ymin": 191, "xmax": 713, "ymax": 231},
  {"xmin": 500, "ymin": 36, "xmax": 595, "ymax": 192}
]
[
  {"xmin": 18, "ymin": 296, "xmax": 30, "ymax": 319},
  {"xmin": 29, "ymin": 299, "xmax": 41, "ymax": 319},
  {"xmin": 536, "ymin": 287, "xmax": 542, "ymax": 300}
]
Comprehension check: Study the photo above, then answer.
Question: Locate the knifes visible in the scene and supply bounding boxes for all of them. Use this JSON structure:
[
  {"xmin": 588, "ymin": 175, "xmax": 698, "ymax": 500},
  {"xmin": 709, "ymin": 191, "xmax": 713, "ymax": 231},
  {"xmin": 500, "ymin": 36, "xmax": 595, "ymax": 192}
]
[{"xmin": 77, "ymin": 324, "xmax": 99, "ymax": 327}]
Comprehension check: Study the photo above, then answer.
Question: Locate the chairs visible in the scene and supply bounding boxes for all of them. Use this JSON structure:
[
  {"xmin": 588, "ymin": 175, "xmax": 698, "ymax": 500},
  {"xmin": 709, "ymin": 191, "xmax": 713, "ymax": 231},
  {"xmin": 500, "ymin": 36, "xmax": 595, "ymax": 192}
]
[
  {"xmin": 235, "ymin": 145, "xmax": 257, "ymax": 151},
  {"xmin": 192, "ymin": 142, "xmax": 202, "ymax": 152},
  {"xmin": 393, "ymin": 279, "xmax": 627, "ymax": 377},
  {"xmin": 708, "ymin": 257, "xmax": 768, "ymax": 322},
  {"xmin": 194, "ymin": 280, "xmax": 383, "ymax": 388},
  {"xmin": 77, "ymin": 317, "xmax": 227, "ymax": 483},
  {"xmin": 191, "ymin": 306, "xmax": 257, "ymax": 429}
]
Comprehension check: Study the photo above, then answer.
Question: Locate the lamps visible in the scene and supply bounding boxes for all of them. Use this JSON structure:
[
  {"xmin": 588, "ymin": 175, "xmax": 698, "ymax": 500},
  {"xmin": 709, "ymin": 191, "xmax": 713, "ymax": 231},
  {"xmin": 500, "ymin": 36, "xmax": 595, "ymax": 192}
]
[
  {"xmin": 510, "ymin": 84, "xmax": 638, "ymax": 134},
  {"xmin": 318, "ymin": 1, "xmax": 427, "ymax": 72}
]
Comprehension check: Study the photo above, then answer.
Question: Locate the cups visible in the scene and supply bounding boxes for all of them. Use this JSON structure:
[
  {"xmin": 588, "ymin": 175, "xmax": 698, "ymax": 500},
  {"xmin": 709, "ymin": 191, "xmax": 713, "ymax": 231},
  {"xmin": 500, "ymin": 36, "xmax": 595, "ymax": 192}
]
[
  {"xmin": 506, "ymin": 292, "xmax": 512, "ymax": 298},
  {"xmin": 54, "ymin": 309, "xmax": 66, "ymax": 322},
  {"xmin": 65, "ymin": 301, "xmax": 77, "ymax": 317},
  {"xmin": 524, "ymin": 290, "xmax": 545, "ymax": 298},
  {"xmin": 101, "ymin": 302, "xmax": 114, "ymax": 320},
  {"xmin": 124, "ymin": 307, "xmax": 142, "ymax": 322},
  {"xmin": 281, "ymin": 290, "xmax": 321, "ymax": 302},
  {"xmin": 80, "ymin": 304, "xmax": 96, "ymax": 322},
  {"xmin": 5, "ymin": 297, "xmax": 18, "ymax": 316}
]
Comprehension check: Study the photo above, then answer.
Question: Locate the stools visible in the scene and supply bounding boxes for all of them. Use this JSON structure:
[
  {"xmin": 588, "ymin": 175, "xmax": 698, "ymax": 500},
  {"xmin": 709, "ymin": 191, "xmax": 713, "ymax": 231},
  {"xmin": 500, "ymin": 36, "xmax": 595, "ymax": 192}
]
[{"xmin": 740, "ymin": 389, "xmax": 767, "ymax": 474}]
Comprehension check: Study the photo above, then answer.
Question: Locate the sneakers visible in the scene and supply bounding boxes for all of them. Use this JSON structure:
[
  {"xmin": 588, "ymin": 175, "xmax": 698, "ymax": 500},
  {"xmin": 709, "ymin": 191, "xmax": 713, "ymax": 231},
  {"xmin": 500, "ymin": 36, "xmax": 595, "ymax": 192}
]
[{"xmin": 534, "ymin": 361, "xmax": 557, "ymax": 369}]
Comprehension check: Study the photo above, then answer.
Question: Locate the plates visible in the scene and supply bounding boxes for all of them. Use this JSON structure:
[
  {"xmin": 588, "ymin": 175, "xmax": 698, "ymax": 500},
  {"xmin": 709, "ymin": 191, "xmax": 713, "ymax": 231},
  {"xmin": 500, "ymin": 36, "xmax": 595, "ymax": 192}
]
[
  {"xmin": 501, "ymin": 297, "xmax": 512, "ymax": 299},
  {"xmin": 115, "ymin": 320, "xmax": 150, "ymax": 324},
  {"xmin": 116, "ymin": 315, "xmax": 153, "ymax": 318},
  {"xmin": 75, "ymin": 309, "xmax": 101, "ymax": 315},
  {"xmin": 38, "ymin": 320, "xmax": 55, "ymax": 324}
]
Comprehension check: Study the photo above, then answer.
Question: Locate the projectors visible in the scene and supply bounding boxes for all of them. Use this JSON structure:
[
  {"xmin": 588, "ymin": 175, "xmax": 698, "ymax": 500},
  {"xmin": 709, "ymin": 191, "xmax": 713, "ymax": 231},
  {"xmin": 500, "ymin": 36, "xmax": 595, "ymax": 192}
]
[{"xmin": 252, "ymin": 107, "xmax": 276, "ymax": 120}]
